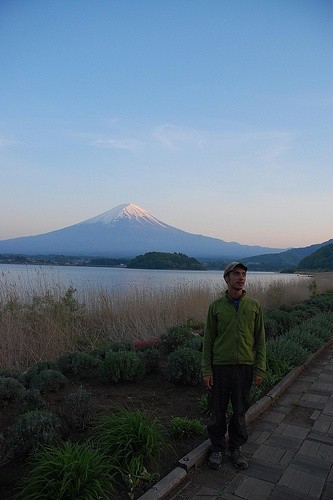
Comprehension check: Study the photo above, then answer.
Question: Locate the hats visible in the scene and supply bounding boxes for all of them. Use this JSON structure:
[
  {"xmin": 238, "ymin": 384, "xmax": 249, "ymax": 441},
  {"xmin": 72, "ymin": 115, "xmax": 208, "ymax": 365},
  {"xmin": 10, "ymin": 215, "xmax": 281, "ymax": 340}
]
[{"xmin": 224, "ymin": 262, "xmax": 248, "ymax": 276}]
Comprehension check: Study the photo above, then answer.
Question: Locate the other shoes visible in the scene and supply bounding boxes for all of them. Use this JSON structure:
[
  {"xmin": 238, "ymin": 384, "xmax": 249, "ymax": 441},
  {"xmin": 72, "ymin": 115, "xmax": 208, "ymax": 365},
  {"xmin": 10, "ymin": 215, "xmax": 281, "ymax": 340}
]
[
  {"xmin": 208, "ymin": 450, "xmax": 223, "ymax": 469},
  {"xmin": 227, "ymin": 446, "xmax": 248, "ymax": 470}
]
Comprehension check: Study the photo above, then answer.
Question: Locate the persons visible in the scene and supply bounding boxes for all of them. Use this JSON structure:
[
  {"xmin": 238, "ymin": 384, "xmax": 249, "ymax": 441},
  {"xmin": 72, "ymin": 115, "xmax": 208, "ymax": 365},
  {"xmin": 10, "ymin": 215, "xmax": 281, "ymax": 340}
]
[{"xmin": 199, "ymin": 260, "xmax": 267, "ymax": 472}]
